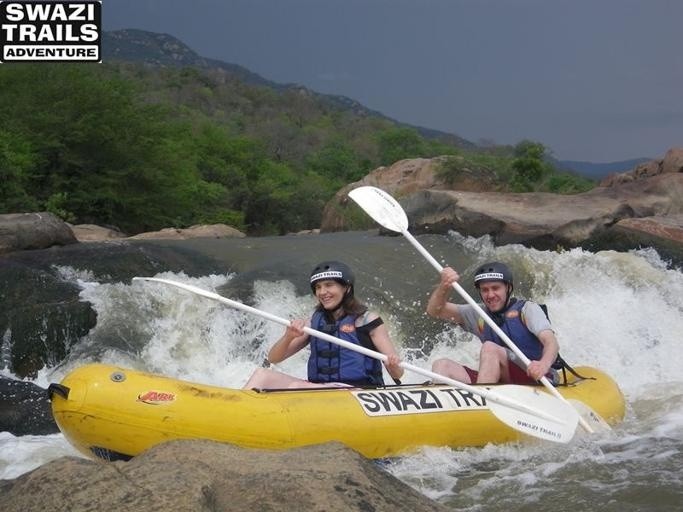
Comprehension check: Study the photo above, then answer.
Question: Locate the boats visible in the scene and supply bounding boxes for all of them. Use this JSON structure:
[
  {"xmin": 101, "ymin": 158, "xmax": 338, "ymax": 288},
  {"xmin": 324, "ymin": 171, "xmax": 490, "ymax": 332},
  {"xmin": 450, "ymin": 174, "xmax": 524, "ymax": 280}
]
[{"xmin": 48, "ymin": 361, "xmax": 625, "ymax": 464}]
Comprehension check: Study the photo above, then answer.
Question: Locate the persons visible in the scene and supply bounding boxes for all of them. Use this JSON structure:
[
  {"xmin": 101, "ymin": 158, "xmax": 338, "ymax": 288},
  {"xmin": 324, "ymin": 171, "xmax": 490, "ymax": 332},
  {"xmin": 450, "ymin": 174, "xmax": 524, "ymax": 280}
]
[
  {"xmin": 266, "ymin": 261, "xmax": 404, "ymax": 388},
  {"xmin": 425, "ymin": 263, "xmax": 562, "ymax": 389}
]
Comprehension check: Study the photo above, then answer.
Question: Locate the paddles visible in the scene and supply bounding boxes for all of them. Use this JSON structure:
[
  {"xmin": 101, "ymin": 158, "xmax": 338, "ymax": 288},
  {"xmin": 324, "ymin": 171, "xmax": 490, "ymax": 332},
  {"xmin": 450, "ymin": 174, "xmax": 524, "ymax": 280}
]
[
  {"xmin": 132, "ymin": 276, "xmax": 579, "ymax": 442},
  {"xmin": 348, "ymin": 186, "xmax": 616, "ymax": 442}
]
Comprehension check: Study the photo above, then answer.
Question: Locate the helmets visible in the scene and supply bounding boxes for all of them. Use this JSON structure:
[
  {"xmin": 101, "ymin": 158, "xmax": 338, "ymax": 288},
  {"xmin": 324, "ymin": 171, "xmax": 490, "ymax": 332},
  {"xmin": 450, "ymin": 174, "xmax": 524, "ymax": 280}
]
[
  {"xmin": 311, "ymin": 261, "xmax": 354, "ymax": 293},
  {"xmin": 474, "ymin": 263, "xmax": 512, "ymax": 288}
]
